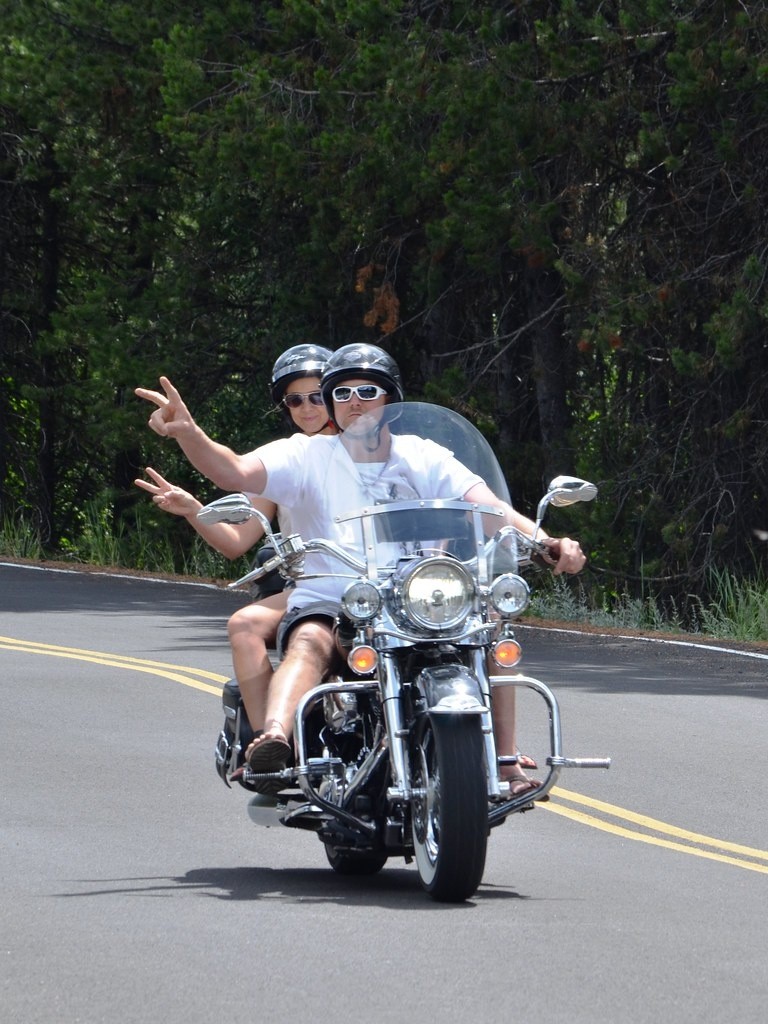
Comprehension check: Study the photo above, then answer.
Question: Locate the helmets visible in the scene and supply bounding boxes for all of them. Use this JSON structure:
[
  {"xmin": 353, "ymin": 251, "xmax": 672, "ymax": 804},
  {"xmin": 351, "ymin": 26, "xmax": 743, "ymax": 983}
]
[
  {"xmin": 268, "ymin": 344, "xmax": 334, "ymax": 415},
  {"xmin": 318, "ymin": 343, "xmax": 403, "ymax": 423}
]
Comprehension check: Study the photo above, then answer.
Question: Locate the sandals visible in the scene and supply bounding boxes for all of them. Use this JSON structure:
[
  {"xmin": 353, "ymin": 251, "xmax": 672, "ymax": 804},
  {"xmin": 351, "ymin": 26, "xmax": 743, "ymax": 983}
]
[
  {"xmin": 499, "ymin": 774, "xmax": 550, "ymax": 802},
  {"xmin": 245, "ymin": 732, "xmax": 291, "ymax": 795}
]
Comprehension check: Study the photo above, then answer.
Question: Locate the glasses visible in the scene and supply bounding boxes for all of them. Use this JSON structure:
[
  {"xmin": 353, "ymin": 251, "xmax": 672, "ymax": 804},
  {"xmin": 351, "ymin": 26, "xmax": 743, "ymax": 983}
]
[
  {"xmin": 332, "ymin": 386, "xmax": 388, "ymax": 403},
  {"xmin": 283, "ymin": 392, "xmax": 325, "ymax": 409}
]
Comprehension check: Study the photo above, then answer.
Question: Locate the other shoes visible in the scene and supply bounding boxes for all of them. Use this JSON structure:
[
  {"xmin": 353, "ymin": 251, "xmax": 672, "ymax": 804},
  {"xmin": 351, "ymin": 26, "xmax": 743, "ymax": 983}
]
[
  {"xmin": 229, "ymin": 764, "xmax": 244, "ymax": 782},
  {"xmin": 516, "ymin": 750, "xmax": 538, "ymax": 770}
]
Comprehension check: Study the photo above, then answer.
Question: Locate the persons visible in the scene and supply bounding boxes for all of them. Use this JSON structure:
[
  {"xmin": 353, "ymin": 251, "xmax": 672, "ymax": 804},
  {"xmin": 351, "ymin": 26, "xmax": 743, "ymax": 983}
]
[
  {"xmin": 135, "ymin": 343, "xmax": 333, "ymax": 780},
  {"xmin": 133, "ymin": 342, "xmax": 587, "ymax": 803}
]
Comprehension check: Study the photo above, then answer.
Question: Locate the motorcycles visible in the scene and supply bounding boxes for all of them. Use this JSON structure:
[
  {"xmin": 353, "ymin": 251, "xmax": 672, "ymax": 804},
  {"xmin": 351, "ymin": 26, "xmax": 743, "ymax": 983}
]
[{"xmin": 196, "ymin": 399, "xmax": 610, "ymax": 903}]
